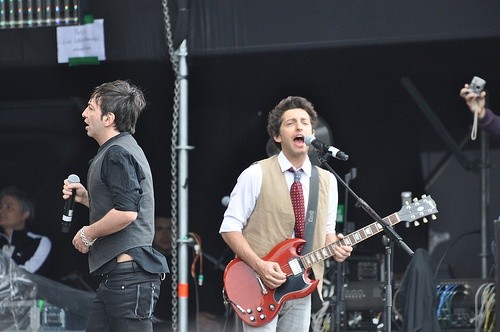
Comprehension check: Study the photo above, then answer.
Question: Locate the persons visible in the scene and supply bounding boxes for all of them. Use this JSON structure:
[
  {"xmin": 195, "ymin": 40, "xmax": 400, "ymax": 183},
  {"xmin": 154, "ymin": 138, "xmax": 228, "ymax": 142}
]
[
  {"xmin": 460, "ymin": 83, "xmax": 500, "ymax": 136},
  {"xmin": 62, "ymin": 80, "xmax": 171, "ymax": 332},
  {"xmin": 219, "ymin": 96, "xmax": 353, "ymax": 332},
  {"xmin": 150, "ymin": 209, "xmax": 222, "ymax": 332},
  {"xmin": 0, "ymin": 185, "xmax": 58, "ymax": 277}
]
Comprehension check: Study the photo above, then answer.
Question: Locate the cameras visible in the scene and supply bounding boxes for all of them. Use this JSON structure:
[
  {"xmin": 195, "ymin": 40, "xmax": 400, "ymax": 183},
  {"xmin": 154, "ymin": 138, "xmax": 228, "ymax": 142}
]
[{"xmin": 468, "ymin": 76, "xmax": 487, "ymax": 98}]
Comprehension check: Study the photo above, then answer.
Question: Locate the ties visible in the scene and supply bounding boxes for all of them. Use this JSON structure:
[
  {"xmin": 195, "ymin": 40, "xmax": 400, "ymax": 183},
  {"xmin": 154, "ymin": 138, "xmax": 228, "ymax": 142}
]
[{"xmin": 288, "ymin": 167, "xmax": 306, "ymax": 254}]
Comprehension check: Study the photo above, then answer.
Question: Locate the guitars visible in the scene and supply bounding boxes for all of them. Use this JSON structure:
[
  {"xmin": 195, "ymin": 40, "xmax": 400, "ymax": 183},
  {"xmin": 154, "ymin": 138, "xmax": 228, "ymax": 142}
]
[{"xmin": 224, "ymin": 195, "xmax": 439, "ymax": 327}]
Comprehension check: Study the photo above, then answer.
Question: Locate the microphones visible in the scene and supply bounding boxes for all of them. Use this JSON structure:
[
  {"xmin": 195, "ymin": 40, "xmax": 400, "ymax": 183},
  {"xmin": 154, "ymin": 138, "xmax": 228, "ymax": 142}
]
[{"xmin": 59, "ymin": 173, "xmax": 80, "ymax": 233}]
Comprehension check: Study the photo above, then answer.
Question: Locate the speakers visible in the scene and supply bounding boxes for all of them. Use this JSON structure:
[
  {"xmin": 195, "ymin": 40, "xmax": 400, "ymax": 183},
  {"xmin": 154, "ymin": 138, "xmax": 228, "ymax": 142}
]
[{"xmin": 304, "ymin": 134, "xmax": 350, "ymax": 161}]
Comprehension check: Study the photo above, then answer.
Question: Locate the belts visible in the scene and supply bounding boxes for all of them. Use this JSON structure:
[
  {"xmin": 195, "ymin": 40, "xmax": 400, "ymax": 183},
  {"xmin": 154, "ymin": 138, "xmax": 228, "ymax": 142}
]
[{"xmin": 102, "ymin": 261, "xmax": 143, "ymax": 276}]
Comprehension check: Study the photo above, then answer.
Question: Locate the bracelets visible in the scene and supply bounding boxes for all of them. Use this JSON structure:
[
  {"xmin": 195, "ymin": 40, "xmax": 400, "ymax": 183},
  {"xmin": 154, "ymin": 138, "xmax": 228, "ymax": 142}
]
[{"xmin": 80, "ymin": 226, "xmax": 97, "ymax": 246}]
